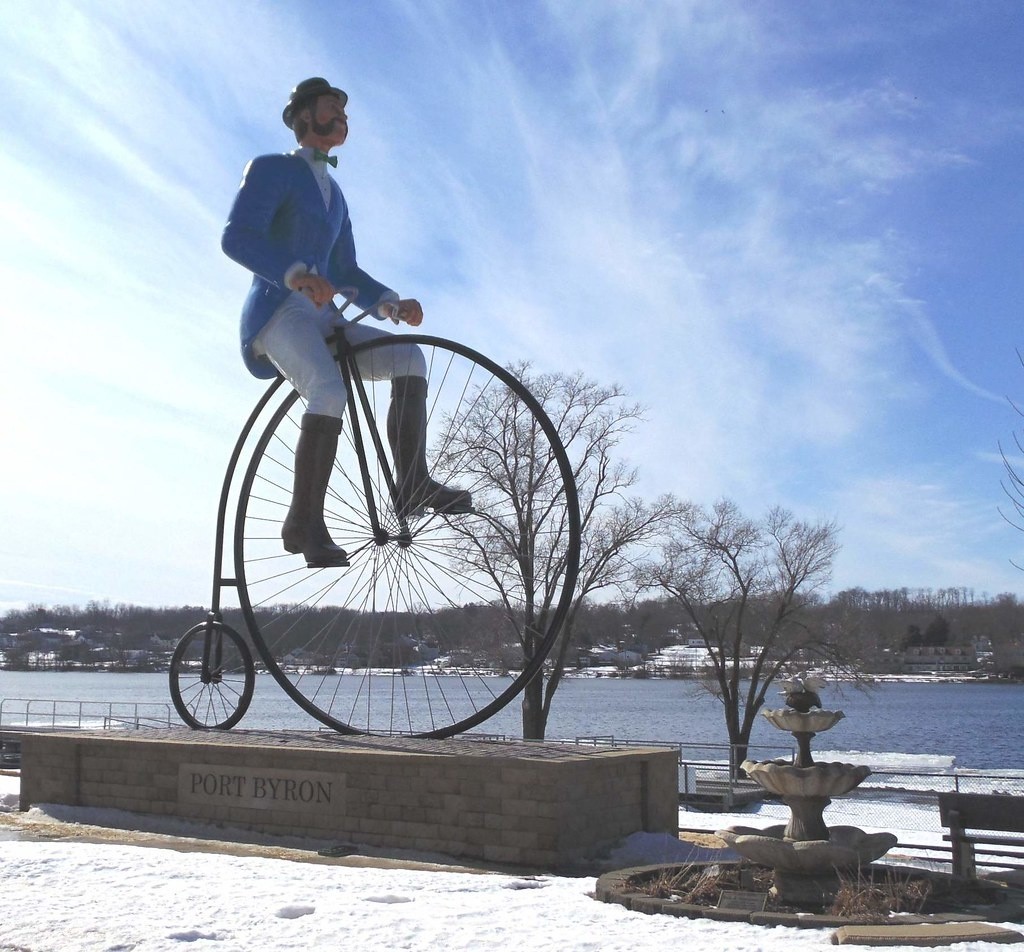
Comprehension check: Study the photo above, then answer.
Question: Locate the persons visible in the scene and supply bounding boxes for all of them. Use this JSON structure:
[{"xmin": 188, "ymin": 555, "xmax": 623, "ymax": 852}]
[{"xmin": 224, "ymin": 77, "xmax": 472, "ymax": 568}]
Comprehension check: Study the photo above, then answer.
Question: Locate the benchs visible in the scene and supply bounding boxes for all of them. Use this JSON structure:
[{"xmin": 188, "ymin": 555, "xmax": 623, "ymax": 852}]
[{"xmin": 937, "ymin": 792, "xmax": 1024, "ymax": 881}]
